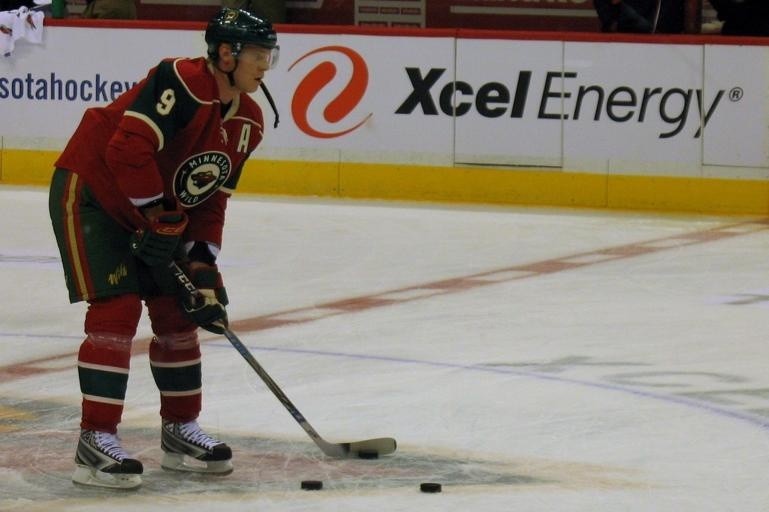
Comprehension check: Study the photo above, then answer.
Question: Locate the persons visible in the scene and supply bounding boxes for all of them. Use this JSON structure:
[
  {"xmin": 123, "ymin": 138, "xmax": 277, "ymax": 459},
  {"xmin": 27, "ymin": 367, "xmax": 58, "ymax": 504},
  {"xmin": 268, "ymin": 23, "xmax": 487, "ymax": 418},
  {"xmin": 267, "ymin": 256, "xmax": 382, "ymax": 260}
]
[{"xmin": 48, "ymin": 6, "xmax": 277, "ymax": 475}]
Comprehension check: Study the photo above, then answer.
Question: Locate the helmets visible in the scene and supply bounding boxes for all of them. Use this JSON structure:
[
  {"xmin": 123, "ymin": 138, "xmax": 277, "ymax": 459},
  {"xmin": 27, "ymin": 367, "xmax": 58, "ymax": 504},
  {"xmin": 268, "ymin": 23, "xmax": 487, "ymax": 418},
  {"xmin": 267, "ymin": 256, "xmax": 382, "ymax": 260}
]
[{"xmin": 205, "ymin": 7, "xmax": 279, "ymax": 70}]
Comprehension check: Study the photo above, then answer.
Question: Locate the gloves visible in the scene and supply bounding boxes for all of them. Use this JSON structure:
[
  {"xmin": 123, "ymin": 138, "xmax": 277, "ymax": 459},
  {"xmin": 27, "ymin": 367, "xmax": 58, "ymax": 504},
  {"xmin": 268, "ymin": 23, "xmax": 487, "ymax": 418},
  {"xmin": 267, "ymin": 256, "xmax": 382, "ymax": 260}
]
[
  {"xmin": 128, "ymin": 195, "xmax": 190, "ymax": 280},
  {"xmin": 177, "ymin": 263, "xmax": 229, "ymax": 335}
]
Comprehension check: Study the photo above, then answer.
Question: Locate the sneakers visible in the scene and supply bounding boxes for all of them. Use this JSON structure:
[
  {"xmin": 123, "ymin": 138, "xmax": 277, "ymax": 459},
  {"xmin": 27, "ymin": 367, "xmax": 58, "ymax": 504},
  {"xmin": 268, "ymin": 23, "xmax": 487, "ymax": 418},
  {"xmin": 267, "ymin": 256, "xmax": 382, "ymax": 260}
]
[
  {"xmin": 74, "ymin": 429, "xmax": 143, "ymax": 474},
  {"xmin": 161, "ymin": 417, "xmax": 232, "ymax": 461}
]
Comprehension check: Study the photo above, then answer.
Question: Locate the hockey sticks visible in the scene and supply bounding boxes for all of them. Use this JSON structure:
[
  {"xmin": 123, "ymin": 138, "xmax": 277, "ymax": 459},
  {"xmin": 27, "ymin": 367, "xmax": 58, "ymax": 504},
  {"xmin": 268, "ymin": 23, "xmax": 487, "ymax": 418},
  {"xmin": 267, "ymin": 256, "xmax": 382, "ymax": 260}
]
[{"xmin": 168, "ymin": 261, "xmax": 397, "ymax": 457}]
